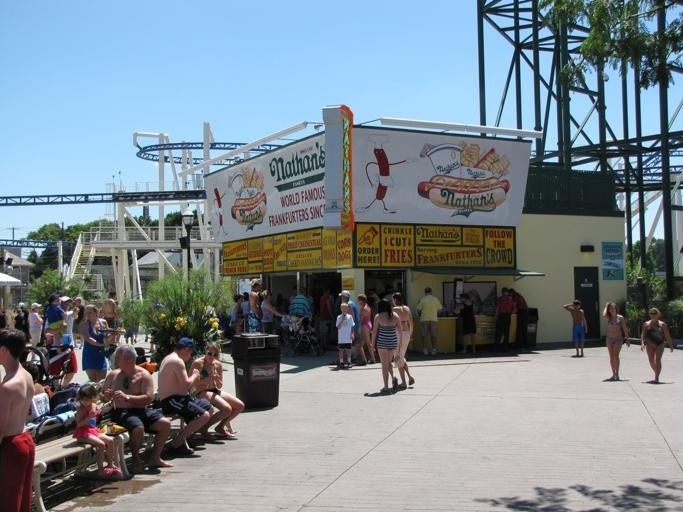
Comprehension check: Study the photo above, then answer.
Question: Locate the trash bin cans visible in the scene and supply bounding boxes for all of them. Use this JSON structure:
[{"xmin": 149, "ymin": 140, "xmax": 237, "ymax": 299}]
[{"xmin": 232, "ymin": 333, "xmax": 280, "ymax": 408}]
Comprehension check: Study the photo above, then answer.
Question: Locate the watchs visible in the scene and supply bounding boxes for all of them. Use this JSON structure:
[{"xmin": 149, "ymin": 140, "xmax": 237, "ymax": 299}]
[{"xmin": 124, "ymin": 395, "xmax": 131, "ymax": 402}]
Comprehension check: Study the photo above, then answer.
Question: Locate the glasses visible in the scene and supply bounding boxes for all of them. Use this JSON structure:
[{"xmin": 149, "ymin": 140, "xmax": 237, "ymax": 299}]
[
  {"xmin": 207, "ymin": 352, "xmax": 217, "ymax": 356},
  {"xmin": 190, "ymin": 339, "xmax": 199, "ymax": 347}
]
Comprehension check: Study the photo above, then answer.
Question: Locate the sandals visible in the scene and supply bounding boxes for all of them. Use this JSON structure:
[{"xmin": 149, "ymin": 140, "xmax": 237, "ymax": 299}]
[
  {"xmin": 381, "ymin": 379, "xmax": 414, "ymax": 394},
  {"xmin": 97, "ymin": 468, "xmax": 113, "ymax": 476},
  {"xmin": 329, "ymin": 356, "xmax": 376, "ymax": 369},
  {"xmin": 107, "ymin": 465, "xmax": 123, "ymax": 477}
]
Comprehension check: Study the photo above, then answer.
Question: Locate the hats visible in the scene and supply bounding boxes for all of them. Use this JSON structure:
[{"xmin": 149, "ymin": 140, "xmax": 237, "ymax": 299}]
[
  {"xmin": 178, "ymin": 337, "xmax": 197, "ymax": 355},
  {"xmin": 338, "ymin": 290, "xmax": 349, "ymax": 297},
  {"xmin": 60, "ymin": 296, "xmax": 71, "ymax": 303},
  {"xmin": 47, "ymin": 294, "xmax": 60, "ymax": 302},
  {"xmin": 31, "ymin": 303, "xmax": 42, "ymax": 309}
]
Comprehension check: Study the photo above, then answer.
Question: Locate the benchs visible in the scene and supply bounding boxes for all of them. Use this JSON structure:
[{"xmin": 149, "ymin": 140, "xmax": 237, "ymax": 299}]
[{"xmin": 29, "ymin": 400, "xmax": 189, "ymax": 511}]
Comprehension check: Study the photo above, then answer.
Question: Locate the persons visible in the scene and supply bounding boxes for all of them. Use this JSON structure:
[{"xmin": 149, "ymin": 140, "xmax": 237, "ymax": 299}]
[
  {"xmin": 452, "ymin": 291, "xmax": 480, "ymax": 358},
  {"xmin": 229, "ymin": 282, "xmax": 398, "ymax": 369},
  {"xmin": 191, "ymin": 343, "xmax": 245, "ymax": 439},
  {"xmin": 508, "ymin": 288, "xmax": 529, "ymax": 347},
  {"xmin": 562, "ymin": 299, "xmax": 588, "ymax": 359},
  {"xmin": 125, "ymin": 296, "xmax": 165, "ymax": 345},
  {"xmin": 0, "ymin": 328, "xmax": 37, "ymax": 511},
  {"xmin": 156, "ymin": 338, "xmax": 212, "ymax": 457},
  {"xmin": 391, "ymin": 292, "xmax": 419, "ymax": 390},
  {"xmin": 602, "ymin": 300, "xmax": 631, "ymax": 382},
  {"xmin": 364, "ymin": 271, "xmax": 406, "ymax": 306},
  {"xmin": 640, "ymin": 307, "xmax": 674, "ymax": 386},
  {"xmin": 73, "ymin": 382, "xmax": 124, "ymax": 481},
  {"xmin": 416, "ymin": 286, "xmax": 445, "ymax": 357},
  {"xmin": 493, "ymin": 287, "xmax": 511, "ymax": 354},
  {"xmin": 4, "ymin": 289, "xmax": 121, "ymax": 387},
  {"xmin": 369, "ymin": 298, "xmax": 405, "ymax": 397},
  {"xmin": 101, "ymin": 343, "xmax": 171, "ymax": 470}
]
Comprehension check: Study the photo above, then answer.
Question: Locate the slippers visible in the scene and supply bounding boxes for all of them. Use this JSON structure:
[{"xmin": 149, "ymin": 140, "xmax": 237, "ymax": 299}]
[
  {"xmin": 170, "ymin": 443, "xmax": 193, "ymax": 454},
  {"xmin": 214, "ymin": 432, "xmax": 236, "ymax": 440}
]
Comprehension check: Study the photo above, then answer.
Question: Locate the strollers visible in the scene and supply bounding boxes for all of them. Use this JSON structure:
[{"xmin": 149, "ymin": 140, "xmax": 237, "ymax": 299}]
[{"xmin": 280, "ymin": 314, "xmax": 318, "ymax": 356}]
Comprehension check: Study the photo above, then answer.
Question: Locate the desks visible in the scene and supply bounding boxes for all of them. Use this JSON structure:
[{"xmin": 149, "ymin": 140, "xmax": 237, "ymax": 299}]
[{"xmin": 414, "ymin": 312, "xmax": 518, "ymax": 356}]
